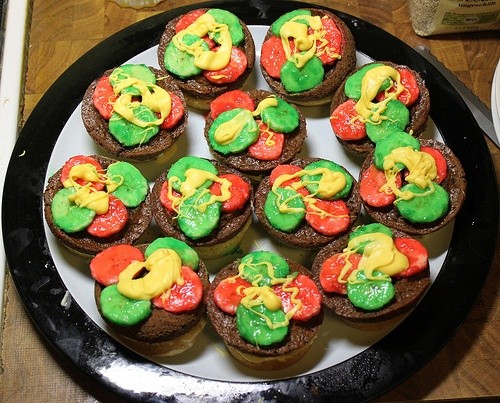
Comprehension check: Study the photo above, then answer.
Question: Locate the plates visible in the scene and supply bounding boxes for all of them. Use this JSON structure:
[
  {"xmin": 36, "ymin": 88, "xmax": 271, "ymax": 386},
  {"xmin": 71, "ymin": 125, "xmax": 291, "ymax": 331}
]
[{"xmin": 1, "ymin": 0, "xmax": 500, "ymax": 403}]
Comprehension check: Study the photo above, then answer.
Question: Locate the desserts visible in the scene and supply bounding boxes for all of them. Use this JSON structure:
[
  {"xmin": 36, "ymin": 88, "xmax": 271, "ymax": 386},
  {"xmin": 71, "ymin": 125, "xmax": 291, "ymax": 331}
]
[{"xmin": 40, "ymin": 9, "xmax": 469, "ymax": 376}]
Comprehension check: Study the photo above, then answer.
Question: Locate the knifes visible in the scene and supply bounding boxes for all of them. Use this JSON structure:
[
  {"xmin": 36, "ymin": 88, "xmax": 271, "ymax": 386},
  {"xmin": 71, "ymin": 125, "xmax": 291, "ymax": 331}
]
[{"xmin": 413, "ymin": 46, "xmax": 500, "ymax": 148}]
